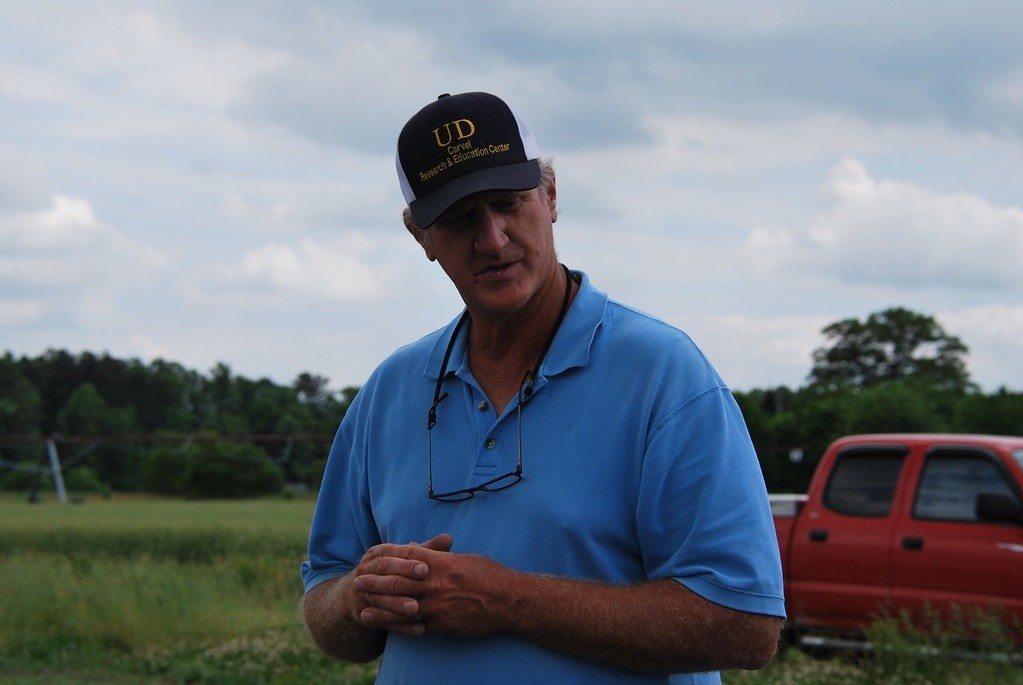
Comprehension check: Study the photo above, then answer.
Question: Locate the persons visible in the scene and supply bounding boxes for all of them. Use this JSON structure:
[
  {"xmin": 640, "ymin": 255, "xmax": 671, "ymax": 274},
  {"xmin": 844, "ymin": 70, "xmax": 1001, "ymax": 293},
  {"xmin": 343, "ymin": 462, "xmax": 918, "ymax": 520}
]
[{"xmin": 299, "ymin": 89, "xmax": 788, "ymax": 684}]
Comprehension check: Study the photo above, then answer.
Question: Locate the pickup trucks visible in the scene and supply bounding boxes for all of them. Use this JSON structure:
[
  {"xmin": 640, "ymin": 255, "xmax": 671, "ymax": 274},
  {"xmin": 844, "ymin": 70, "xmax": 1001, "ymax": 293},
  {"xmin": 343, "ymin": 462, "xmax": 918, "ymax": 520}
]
[{"xmin": 757, "ymin": 431, "xmax": 1023, "ymax": 670}]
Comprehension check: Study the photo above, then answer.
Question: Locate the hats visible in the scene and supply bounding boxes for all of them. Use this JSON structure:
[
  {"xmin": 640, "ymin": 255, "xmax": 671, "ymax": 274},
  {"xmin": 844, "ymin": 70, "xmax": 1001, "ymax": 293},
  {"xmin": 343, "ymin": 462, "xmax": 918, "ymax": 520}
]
[{"xmin": 395, "ymin": 91, "xmax": 542, "ymax": 230}]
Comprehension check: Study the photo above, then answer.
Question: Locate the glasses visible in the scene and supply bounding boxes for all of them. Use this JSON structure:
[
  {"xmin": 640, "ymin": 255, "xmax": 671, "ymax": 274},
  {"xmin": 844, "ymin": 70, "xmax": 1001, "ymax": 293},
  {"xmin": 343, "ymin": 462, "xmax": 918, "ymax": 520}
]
[{"xmin": 426, "ymin": 370, "xmax": 532, "ymax": 504}]
